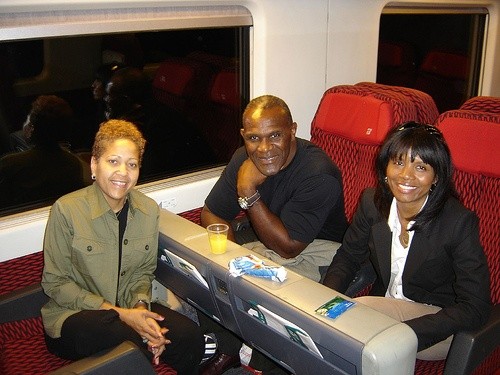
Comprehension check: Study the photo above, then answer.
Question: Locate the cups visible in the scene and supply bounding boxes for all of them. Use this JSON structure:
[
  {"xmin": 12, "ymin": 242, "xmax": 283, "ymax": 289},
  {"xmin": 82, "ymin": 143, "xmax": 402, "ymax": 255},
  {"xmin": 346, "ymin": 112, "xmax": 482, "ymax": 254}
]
[{"xmin": 207, "ymin": 224, "xmax": 229, "ymax": 254}]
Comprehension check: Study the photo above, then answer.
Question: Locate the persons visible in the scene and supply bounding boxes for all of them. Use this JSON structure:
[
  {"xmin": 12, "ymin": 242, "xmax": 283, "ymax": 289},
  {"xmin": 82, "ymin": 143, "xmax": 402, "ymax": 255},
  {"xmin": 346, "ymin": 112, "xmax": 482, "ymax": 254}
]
[
  {"xmin": 0, "ymin": 96, "xmax": 84, "ymax": 206},
  {"xmin": 25, "ymin": 66, "xmax": 63, "ymax": 100},
  {"xmin": 41, "ymin": 118, "xmax": 205, "ymax": 375},
  {"xmin": 90, "ymin": 60, "xmax": 218, "ymax": 179},
  {"xmin": 199, "ymin": 95, "xmax": 349, "ymax": 375},
  {"xmin": 324, "ymin": 121, "xmax": 491, "ymax": 361}
]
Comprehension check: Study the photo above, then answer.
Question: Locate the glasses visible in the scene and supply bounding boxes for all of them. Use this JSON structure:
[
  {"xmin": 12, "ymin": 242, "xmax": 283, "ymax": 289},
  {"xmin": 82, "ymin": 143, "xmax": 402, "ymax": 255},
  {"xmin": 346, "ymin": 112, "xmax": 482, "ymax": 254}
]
[{"xmin": 392, "ymin": 122, "xmax": 443, "ymax": 148}]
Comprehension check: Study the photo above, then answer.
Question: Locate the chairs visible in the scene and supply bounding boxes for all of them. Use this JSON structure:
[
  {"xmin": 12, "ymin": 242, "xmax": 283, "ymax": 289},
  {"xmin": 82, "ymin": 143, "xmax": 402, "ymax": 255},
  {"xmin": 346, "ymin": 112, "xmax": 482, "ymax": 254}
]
[
  {"xmin": 0, "ymin": 282, "xmax": 156, "ymax": 375},
  {"xmin": 176, "ymin": 82, "xmax": 500, "ymax": 375}
]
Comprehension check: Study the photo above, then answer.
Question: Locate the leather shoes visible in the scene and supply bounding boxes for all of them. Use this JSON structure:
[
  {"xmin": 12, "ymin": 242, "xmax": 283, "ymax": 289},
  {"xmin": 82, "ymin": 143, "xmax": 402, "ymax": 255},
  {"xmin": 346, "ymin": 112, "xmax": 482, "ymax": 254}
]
[{"xmin": 197, "ymin": 348, "xmax": 241, "ymax": 375}]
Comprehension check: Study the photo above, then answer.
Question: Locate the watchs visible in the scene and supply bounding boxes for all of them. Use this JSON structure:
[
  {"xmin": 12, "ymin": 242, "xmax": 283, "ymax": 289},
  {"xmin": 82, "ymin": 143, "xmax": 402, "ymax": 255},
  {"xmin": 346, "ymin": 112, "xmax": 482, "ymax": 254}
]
[{"xmin": 237, "ymin": 190, "xmax": 262, "ymax": 210}]
